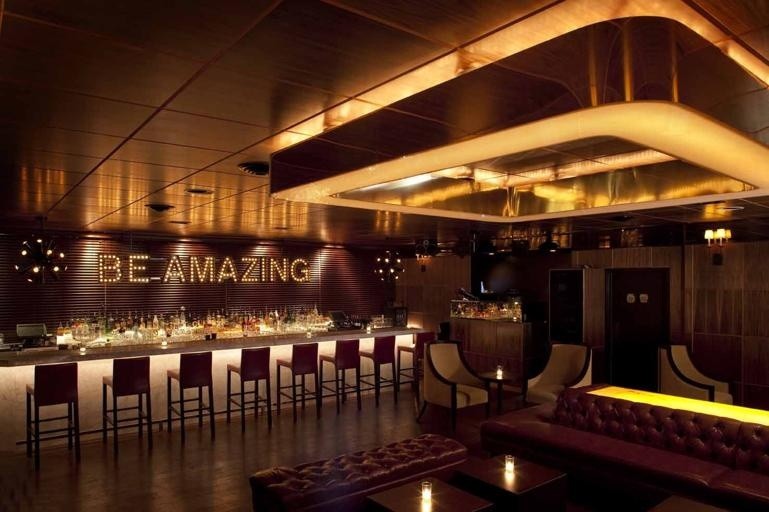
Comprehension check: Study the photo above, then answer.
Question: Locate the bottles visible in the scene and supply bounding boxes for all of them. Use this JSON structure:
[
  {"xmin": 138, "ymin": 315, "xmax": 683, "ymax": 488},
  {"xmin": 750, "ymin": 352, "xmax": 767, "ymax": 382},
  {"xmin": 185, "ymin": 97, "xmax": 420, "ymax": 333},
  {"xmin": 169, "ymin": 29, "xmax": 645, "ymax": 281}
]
[
  {"xmin": 57, "ymin": 302, "xmax": 332, "ymax": 340},
  {"xmin": 346, "ymin": 314, "xmax": 393, "ymax": 329}
]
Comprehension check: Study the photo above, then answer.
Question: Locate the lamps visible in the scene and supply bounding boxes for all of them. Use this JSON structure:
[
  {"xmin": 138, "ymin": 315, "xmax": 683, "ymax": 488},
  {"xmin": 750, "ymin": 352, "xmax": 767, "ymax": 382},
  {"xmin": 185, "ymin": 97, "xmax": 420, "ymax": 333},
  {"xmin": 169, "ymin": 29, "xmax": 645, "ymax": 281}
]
[
  {"xmin": 13, "ymin": 216, "xmax": 70, "ymax": 284},
  {"xmin": 372, "ymin": 249, "xmax": 407, "ymax": 286},
  {"xmin": 703, "ymin": 228, "xmax": 733, "ymax": 256}
]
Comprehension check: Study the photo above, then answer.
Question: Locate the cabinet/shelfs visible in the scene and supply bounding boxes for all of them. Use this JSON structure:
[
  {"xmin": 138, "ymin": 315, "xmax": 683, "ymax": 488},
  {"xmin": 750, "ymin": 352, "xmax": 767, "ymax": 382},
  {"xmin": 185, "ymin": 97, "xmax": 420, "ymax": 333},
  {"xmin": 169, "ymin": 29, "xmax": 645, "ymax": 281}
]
[{"xmin": 448, "ymin": 318, "xmax": 532, "ymax": 360}]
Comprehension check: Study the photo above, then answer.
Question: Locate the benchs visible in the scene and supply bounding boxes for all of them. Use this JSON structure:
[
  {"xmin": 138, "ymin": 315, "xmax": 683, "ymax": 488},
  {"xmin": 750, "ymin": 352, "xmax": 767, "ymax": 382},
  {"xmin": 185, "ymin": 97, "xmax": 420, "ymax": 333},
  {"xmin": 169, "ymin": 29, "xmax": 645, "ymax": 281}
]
[{"xmin": 249, "ymin": 433, "xmax": 467, "ymax": 512}]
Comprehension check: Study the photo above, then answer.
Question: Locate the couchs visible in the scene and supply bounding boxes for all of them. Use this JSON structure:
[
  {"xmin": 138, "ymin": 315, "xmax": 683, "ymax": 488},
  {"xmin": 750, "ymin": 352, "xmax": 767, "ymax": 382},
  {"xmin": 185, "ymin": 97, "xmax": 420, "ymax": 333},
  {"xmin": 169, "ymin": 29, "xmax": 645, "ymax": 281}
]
[{"xmin": 481, "ymin": 383, "xmax": 766, "ymax": 512}]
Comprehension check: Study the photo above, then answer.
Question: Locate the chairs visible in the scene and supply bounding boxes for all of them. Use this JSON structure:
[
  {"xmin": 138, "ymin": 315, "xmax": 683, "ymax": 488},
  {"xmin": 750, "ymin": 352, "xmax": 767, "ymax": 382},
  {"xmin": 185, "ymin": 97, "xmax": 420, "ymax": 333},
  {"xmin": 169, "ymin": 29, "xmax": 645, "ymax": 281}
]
[
  {"xmin": 659, "ymin": 346, "xmax": 733, "ymax": 406},
  {"xmin": 415, "ymin": 340, "xmax": 593, "ymax": 432}
]
